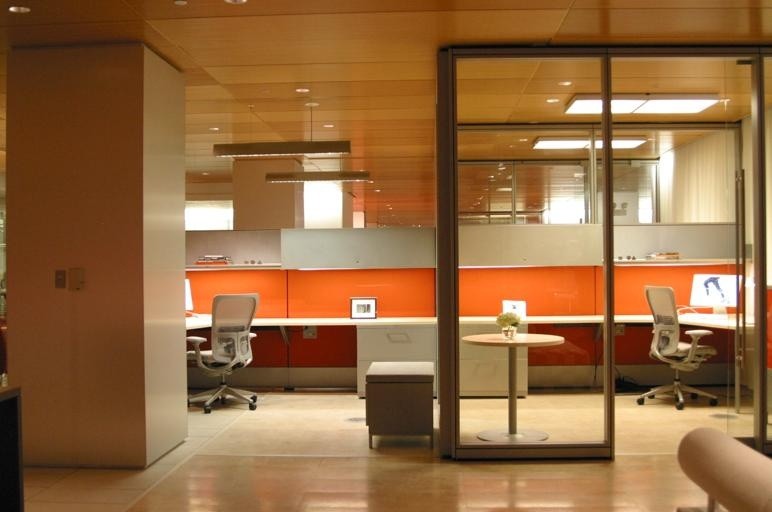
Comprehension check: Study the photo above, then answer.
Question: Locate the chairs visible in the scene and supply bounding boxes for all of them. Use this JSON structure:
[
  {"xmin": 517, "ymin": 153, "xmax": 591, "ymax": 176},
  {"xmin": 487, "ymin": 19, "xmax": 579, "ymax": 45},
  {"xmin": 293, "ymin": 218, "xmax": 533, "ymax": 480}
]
[
  {"xmin": 637, "ymin": 286, "xmax": 718, "ymax": 409},
  {"xmin": 187, "ymin": 294, "xmax": 261, "ymax": 415}
]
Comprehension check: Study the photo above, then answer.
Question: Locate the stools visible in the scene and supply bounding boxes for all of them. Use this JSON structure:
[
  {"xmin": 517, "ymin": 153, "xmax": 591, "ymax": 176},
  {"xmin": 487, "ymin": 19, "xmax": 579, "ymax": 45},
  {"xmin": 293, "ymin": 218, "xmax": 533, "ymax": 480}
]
[{"xmin": 366, "ymin": 362, "xmax": 434, "ymax": 449}]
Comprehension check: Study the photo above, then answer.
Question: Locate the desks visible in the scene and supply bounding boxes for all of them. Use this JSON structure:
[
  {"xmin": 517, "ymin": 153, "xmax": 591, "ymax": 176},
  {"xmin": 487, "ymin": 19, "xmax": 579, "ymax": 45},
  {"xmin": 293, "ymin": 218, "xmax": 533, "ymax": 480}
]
[
  {"xmin": 462, "ymin": 333, "xmax": 565, "ymax": 444},
  {"xmin": 186, "ymin": 316, "xmax": 760, "ymax": 398}
]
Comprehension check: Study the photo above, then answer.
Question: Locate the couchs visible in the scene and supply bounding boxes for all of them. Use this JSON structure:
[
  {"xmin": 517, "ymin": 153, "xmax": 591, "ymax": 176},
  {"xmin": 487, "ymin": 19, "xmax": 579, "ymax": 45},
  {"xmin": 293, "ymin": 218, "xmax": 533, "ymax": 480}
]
[{"xmin": 674, "ymin": 427, "xmax": 772, "ymax": 512}]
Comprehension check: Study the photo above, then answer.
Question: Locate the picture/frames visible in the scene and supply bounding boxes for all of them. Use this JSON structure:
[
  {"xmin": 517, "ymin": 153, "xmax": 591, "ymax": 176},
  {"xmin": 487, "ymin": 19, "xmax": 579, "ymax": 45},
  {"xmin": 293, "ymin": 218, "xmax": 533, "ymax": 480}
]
[{"xmin": 350, "ymin": 297, "xmax": 377, "ymax": 321}]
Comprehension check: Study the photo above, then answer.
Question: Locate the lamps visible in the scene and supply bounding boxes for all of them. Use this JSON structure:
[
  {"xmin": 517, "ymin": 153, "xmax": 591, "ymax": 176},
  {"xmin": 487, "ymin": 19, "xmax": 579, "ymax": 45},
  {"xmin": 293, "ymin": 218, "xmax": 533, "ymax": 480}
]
[
  {"xmin": 529, "ymin": 94, "xmax": 719, "ymax": 151},
  {"xmin": 212, "ymin": 143, "xmax": 371, "ymax": 183}
]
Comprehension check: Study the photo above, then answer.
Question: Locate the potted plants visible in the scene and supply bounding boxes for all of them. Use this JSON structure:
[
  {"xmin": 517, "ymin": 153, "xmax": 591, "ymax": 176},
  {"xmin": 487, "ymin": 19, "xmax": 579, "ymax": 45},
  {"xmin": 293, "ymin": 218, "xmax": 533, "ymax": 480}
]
[{"xmin": 499, "ymin": 311, "xmax": 520, "ymax": 338}]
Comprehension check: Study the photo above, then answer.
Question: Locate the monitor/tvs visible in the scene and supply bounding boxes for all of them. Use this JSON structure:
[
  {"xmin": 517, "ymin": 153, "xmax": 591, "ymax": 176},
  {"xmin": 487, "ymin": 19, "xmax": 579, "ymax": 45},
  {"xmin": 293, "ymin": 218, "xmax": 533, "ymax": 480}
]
[{"xmin": 689, "ymin": 273, "xmax": 742, "ymax": 315}]
